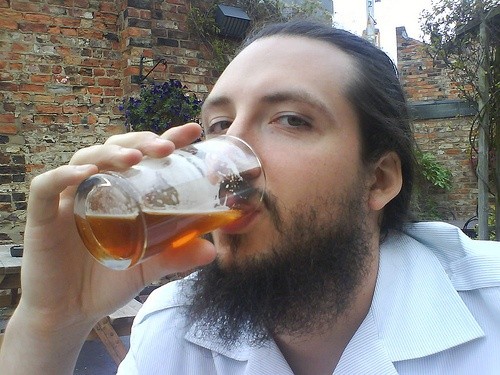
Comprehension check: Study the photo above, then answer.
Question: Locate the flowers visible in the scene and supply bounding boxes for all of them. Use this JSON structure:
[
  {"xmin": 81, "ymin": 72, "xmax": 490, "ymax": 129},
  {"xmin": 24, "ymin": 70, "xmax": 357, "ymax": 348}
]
[{"xmin": 117, "ymin": 77, "xmax": 204, "ymax": 143}]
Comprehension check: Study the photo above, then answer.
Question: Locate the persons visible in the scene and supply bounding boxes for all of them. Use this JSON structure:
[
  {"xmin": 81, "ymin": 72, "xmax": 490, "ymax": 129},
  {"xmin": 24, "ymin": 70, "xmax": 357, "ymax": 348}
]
[{"xmin": 0, "ymin": 18, "xmax": 500, "ymax": 375}]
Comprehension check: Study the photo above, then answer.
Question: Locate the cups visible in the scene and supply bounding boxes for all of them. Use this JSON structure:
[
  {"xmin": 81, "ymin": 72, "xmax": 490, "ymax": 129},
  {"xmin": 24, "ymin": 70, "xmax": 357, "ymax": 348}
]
[{"xmin": 74, "ymin": 135, "xmax": 265, "ymax": 270}]
